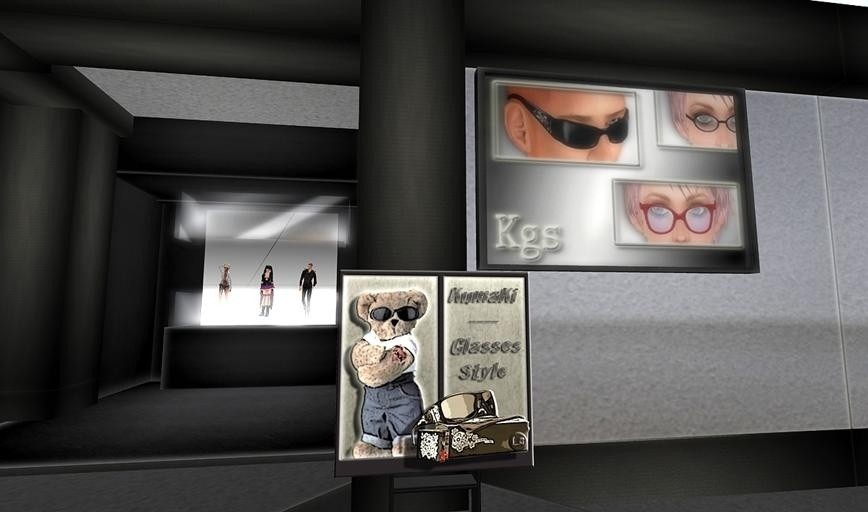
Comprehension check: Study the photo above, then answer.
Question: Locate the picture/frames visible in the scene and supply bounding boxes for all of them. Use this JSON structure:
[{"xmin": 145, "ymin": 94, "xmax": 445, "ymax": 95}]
[
  {"xmin": 652, "ymin": 90, "xmax": 740, "ymax": 154},
  {"xmin": 610, "ymin": 177, "xmax": 746, "ymax": 252},
  {"xmin": 491, "ymin": 80, "xmax": 643, "ymax": 169},
  {"xmin": 474, "ymin": 66, "xmax": 762, "ymax": 273},
  {"xmin": 337, "ymin": 271, "xmax": 534, "ymax": 477}
]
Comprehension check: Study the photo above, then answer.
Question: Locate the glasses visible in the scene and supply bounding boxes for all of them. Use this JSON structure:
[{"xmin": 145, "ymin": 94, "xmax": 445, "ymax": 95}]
[
  {"xmin": 641, "ymin": 200, "xmax": 716, "ymax": 234},
  {"xmin": 686, "ymin": 112, "xmax": 737, "ymax": 134},
  {"xmin": 508, "ymin": 93, "xmax": 629, "ymax": 149},
  {"xmin": 412, "ymin": 390, "xmax": 496, "ymax": 447}
]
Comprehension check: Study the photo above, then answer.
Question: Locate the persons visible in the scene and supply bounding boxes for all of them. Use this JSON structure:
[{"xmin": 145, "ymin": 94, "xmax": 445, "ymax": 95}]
[
  {"xmin": 219, "ymin": 264, "xmax": 232, "ymax": 297},
  {"xmin": 299, "ymin": 263, "xmax": 317, "ymax": 313},
  {"xmin": 666, "ymin": 92, "xmax": 737, "ymax": 149},
  {"xmin": 624, "ymin": 185, "xmax": 732, "ymax": 245},
  {"xmin": 259, "ymin": 265, "xmax": 274, "ymax": 317},
  {"xmin": 502, "ymin": 86, "xmax": 627, "ymax": 162}
]
